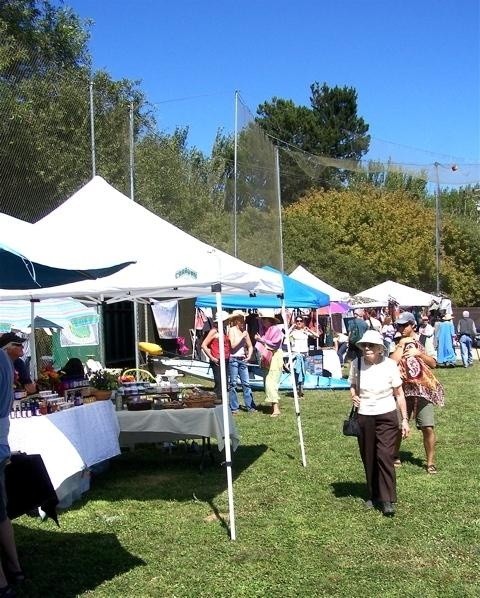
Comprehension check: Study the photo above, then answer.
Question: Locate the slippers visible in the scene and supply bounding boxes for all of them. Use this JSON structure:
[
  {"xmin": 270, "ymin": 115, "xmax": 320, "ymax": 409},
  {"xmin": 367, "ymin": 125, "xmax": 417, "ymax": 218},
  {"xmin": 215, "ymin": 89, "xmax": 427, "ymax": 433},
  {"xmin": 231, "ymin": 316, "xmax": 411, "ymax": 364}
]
[{"xmin": 270, "ymin": 412, "xmax": 280, "ymax": 418}]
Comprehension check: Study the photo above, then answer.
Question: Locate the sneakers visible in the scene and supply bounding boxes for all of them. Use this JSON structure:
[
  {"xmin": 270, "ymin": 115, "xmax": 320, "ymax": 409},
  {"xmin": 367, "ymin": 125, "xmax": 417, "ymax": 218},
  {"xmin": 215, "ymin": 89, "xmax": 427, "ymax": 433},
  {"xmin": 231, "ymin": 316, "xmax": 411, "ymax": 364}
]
[
  {"xmin": 394, "ymin": 457, "xmax": 402, "ymax": 468},
  {"xmin": 366, "ymin": 500, "xmax": 377, "ymax": 510},
  {"xmin": 377, "ymin": 501, "xmax": 395, "ymax": 516},
  {"xmin": 427, "ymin": 465, "xmax": 438, "ymax": 474}
]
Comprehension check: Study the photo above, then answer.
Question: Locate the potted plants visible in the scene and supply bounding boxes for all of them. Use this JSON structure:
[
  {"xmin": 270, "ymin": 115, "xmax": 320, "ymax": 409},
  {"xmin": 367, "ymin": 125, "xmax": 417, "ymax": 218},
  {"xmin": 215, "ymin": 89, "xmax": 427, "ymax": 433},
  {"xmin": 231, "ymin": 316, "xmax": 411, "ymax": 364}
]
[{"xmin": 88, "ymin": 368, "xmax": 122, "ymax": 400}]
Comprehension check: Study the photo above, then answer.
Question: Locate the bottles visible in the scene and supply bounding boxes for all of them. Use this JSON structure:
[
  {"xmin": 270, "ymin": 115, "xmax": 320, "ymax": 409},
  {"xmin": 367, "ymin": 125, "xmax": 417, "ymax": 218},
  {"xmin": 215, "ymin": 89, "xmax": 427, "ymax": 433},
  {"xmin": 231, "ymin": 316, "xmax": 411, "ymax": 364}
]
[
  {"xmin": 66, "ymin": 391, "xmax": 72, "ymax": 403},
  {"xmin": 22, "ymin": 389, "xmax": 29, "ymax": 398},
  {"xmin": 20, "ymin": 396, "xmax": 46, "ymax": 418},
  {"xmin": 15, "ymin": 405, "xmax": 21, "ymax": 418},
  {"xmin": 115, "ymin": 392, "xmax": 124, "ymax": 411},
  {"xmin": 76, "ymin": 390, "xmax": 81, "ymax": 406},
  {"xmin": 14, "ymin": 389, "xmax": 22, "ymax": 399},
  {"xmin": 74, "ymin": 391, "xmax": 76, "ymax": 406},
  {"xmin": 80, "ymin": 396, "xmax": 83, "ymax": 405},
  {"xmin": 10, "ymin": 407, "xmax": 15, "ymax": 419},
  {"xmin": 47, "ymin": 401, "xmax": 58, "ymax": 413},
  {"xmin": 84, "ymin": 395, "xmax": 97, "ymax": 403}
]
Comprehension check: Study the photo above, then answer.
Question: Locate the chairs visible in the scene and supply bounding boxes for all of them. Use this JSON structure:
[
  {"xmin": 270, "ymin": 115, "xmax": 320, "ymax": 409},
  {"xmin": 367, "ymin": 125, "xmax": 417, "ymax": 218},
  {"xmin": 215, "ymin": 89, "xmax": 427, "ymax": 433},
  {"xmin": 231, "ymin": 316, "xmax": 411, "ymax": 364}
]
[{"xmin": 122, "ymin": 368, "xmax": 159, "ymax": 383}]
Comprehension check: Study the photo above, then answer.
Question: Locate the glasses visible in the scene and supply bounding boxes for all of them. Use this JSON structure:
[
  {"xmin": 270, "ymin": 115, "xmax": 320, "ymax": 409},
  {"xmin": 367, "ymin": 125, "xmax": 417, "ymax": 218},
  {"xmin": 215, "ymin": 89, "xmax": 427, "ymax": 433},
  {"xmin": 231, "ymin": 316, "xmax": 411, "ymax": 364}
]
[
  {"xmin": 296, "ymin": 320, "xmax": 303, "ymax": 322},
  {"xmin": 398, "ymin": 323, "xmax": 414, "ymax": 327},
  {"xmin": 361, "ymin": 343, "xmax": 374, "ymax": 348}
]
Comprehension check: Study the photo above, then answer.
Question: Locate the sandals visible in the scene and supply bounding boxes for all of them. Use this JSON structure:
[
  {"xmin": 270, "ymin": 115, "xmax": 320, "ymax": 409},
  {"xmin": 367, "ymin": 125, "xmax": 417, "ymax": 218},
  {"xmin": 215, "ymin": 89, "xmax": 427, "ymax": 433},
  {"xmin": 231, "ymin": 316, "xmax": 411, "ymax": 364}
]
[
  {"xmin": 0, "ymin": 584, "xmax": 17, "ymax": 598},
  {"xmin": 6, "ymin": 570, "xmax": 25, "ymax": 584}
]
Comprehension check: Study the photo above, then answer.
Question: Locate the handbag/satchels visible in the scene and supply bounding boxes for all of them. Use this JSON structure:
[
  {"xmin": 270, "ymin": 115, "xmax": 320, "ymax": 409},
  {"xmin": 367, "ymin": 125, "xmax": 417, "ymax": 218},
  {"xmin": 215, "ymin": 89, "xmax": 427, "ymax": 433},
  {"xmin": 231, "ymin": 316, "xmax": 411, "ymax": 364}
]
[
  {"xmin": 369, "ymin": 326, "xmax": 375, "ymax": 330},
  {"xmin": 314, "ymin": 334, "xmax": 324, "ymax": 347},
  {"xmin": 208, "ymin": 317, "xmax": 214, "ymax": 328},
  {"xmin": 195, "ymin": 316, "xmax": 204, "ymax": 330},
  {"xmin": 326, "ymin": 334, "xmax": 333, "ymax": 347},
  {"xmin": 343, "ymin": 418, "xmax": 361, "ymax": 437},
  {"xmin": 204, "ymin": 320, "xmax": 211, "ymax": 332}
]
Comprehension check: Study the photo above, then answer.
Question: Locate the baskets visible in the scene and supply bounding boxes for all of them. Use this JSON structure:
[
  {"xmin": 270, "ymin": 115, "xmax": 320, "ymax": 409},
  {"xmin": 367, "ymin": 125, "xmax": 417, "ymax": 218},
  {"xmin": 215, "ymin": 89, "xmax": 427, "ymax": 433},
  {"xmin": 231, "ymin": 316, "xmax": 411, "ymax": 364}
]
[
  {"xmin": 182, "ymin": 396, "xmax": 214, "ymax": 408},
  {"xmin": 162, "ymin": 401, "xmax": 184, "ymax": 408}
]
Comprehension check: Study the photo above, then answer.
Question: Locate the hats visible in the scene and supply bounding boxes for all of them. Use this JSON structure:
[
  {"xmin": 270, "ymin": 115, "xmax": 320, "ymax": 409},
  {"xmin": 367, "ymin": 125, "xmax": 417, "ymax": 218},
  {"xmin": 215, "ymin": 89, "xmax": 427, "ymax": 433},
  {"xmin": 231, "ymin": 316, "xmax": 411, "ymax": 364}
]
[
  {"xmin": 422, "ymin": 316, "xmax": 428, "ymax": 320},
  {"xmin": 463, "ymin": 311, "xmax": 469, "ymax": 318},
  {"xmin": 259, "ymin": 310, "xmax": 281, "ymax": 323},
  {"xmin": 213, "ymin": 311, "xmax": 230, "ymax": 322},
  {"xmin": 230, "ymin": 310, "xmax": 249, "ymax": 319},
  {"xmin": 442, "ymin": 314, "xmax": 454, "ymax": 320},
  {"xmin": 355, "ymin": 330, "xmax": 386, "ymax": 350},
  {"xmin": 0, "ymin": 333, "xmax": 25, "ymax": 350},
  {"xmin": 354, "ymin": 309, "xmax": 364, "ymax": 317},
  {"xmin": 396, "ymin": 312, "xmax": 415, "ymax": 325}
]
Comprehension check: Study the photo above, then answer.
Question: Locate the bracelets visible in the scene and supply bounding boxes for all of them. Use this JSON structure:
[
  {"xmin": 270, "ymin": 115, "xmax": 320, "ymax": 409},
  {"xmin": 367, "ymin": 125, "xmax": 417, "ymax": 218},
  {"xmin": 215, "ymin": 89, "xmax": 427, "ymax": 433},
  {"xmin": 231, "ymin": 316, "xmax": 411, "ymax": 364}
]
[{"xmin": 401, "ymin": 418, "xmax": 409, "ymax": 422}]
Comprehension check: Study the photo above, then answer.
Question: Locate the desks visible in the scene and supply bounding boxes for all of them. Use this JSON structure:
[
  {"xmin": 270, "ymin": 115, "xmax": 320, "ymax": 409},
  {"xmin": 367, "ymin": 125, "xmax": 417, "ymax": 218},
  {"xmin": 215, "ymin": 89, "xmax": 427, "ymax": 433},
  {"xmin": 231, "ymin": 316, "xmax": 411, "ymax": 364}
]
[
  {"xmin": 0, "ymin": 399, "xmax": 122, "ymax": 511},
  {"xmin": 111, "ymin": 402, "xmax": 227, "ymax": 473}
]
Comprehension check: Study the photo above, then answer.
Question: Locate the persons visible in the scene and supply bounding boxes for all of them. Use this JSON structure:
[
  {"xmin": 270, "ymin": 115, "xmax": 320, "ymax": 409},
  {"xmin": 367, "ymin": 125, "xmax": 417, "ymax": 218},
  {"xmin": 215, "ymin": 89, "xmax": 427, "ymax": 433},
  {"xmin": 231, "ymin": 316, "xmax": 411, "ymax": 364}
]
[
  {"xmin": 0, "ymin": 348, "xmax": 26, "ymax": 598},
  {"xmin": 388, "ymin": 311, "xmax": 444, "ymax": 474},
  {"xmin": 343, "ymin": 329, "xmax": 410, "ymax": 516},
  {"xmin": 200, "ymin": 308, "xmax": 477, "ymax": 418},
  {"xmin": 0, "ymin": 332, "xmax": 36, "ymax": 395}
]
